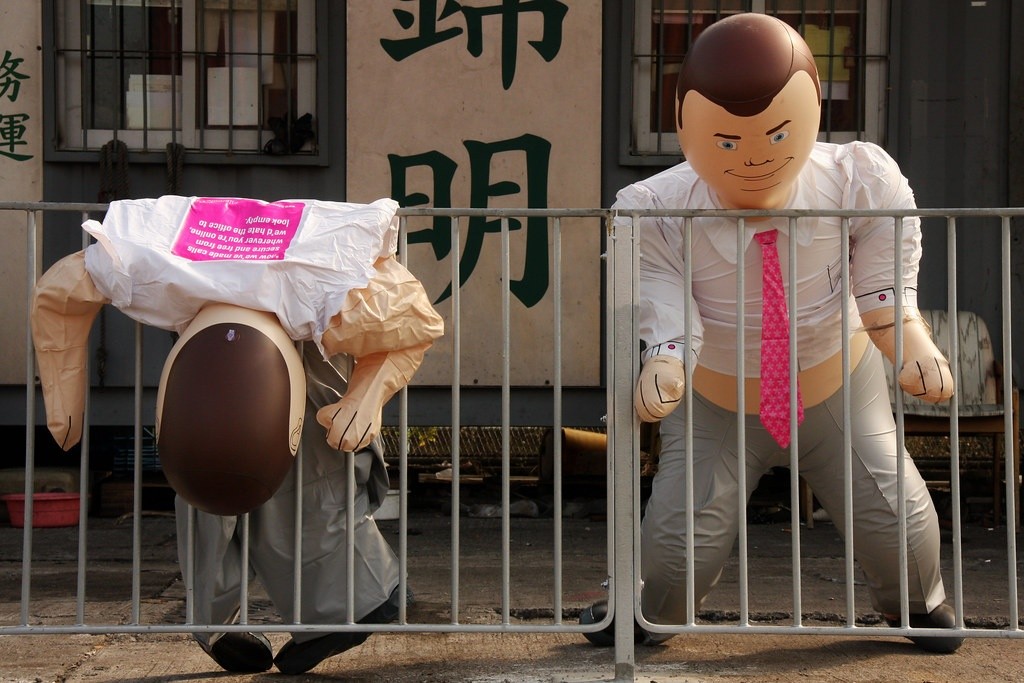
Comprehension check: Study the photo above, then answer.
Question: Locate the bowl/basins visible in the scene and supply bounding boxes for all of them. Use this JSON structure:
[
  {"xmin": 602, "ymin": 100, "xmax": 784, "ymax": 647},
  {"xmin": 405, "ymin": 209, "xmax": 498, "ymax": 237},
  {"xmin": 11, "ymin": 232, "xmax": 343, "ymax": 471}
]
[{"xmin": 0, "ymin": 492, "xmax": 81, "ymax": 528}]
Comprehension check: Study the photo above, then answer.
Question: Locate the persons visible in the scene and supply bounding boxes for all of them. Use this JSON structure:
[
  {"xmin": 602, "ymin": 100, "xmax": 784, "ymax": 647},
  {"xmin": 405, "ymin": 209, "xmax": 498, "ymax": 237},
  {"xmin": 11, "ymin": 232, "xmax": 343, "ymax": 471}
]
[
  {"xmin": 577, "ymin": 11, "xmax": 963, "ymax": 652},
  {"xmin": 31, "ymin": 193, "xmax": 445, "ymax": 680}
]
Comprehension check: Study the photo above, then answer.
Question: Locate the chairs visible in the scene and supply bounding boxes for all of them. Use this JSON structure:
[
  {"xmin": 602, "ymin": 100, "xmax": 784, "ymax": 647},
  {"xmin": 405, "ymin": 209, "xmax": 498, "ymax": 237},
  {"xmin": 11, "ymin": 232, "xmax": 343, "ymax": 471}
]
[{"xmin": 801, "ymin": 307, "xmax": 1022, "ymax": 535}]
